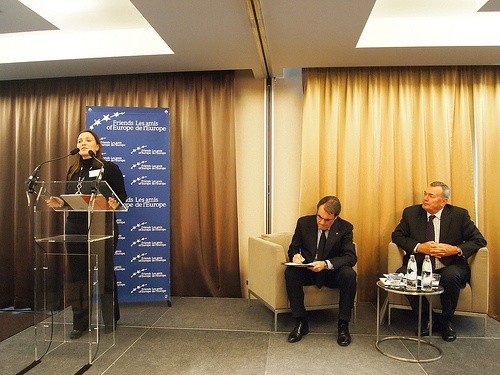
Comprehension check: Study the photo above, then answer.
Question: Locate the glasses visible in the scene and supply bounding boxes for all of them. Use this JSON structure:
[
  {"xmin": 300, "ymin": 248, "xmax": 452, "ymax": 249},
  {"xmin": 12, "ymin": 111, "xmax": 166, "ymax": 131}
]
[{"xmin": 316, "ymin": 215, "xmax": 334, "ymax": 221}]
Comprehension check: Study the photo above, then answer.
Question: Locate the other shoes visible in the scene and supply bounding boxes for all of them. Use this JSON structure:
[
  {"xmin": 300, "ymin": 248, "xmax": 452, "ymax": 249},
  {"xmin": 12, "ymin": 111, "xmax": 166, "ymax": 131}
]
[
  {"xmin": 104, "ymin": 322, "xmax": 116, "ymax": 334},
  {"xmin": 70, "ymin": 326, "xmax": 89, "ymax": 339}
]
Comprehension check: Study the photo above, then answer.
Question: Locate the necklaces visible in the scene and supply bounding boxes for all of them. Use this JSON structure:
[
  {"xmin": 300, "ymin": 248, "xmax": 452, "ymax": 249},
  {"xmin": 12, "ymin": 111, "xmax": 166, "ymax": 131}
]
[{"xmin": 76, "ymin": 165, "xmax": 92, "ymax": 195}]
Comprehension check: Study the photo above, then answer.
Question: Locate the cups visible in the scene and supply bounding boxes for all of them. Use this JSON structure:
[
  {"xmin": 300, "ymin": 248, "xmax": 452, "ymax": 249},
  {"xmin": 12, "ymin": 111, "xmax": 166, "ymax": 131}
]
[{"xmin": 431, "ymin": 273, "xmax": 441, "ymax": 290}]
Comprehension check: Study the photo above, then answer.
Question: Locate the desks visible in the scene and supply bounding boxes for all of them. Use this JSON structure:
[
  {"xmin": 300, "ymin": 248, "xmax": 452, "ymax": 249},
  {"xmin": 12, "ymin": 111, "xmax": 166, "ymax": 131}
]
[{"xmin": 374, "ymin": 280, "xmax": 444, "ymax": 362}]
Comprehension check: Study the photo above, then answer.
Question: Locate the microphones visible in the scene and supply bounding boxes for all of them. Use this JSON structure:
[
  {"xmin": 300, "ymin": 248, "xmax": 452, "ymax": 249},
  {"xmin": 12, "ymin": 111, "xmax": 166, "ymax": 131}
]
[
  {"xmin": 88, "ymin": 150, "xmax": 104, "ymax": 180},
  {"xmin": 28, "ymin": 148, "xmax": 80, "ymax": 181}
]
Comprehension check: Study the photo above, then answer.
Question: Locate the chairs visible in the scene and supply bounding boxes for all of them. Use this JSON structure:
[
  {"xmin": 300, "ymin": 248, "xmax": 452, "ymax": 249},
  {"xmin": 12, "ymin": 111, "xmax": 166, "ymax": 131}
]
[
  {"xmin": 385, "ymin": 240, "xmax": 489, "ymax": 335},
  {"xmin": 246, "ymin": 233, "xmax": 358, "ymax": 331}
]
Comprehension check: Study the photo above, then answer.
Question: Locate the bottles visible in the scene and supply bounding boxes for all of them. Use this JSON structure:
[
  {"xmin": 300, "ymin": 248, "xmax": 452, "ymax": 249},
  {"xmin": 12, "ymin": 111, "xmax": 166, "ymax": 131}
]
[
  {"xmin": 421, "ymin": 255, "xmax": 433, "ymax": 292},
  {"xmin": 405, "ymin": 255, "xmax": 418, "ymax": 292}
]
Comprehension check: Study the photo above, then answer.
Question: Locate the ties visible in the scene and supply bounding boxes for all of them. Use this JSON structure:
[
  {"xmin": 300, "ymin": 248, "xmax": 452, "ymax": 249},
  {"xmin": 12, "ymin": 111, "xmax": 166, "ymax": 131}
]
[
  {"xmin": 317, "ymin": 229, "xmax": 328, "ymax": 261},
  {"xmin": 427, "ymin": 215, "xmax": 436, "ymax": 273}
]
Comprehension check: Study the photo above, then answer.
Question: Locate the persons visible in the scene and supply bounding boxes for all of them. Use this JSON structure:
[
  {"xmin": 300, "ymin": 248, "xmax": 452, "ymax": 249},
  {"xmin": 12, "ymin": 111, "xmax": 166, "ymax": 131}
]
[
  {"xmin": 45, "ymin": 130, "xmax": 128, "ymax": 341},
  {"xmin": 392, "ymin": 181, "xmax": 487, "ymax": 344},
  {"xmin": 285, "ymin": 196, "xmax": 358, "ymax": 346}
]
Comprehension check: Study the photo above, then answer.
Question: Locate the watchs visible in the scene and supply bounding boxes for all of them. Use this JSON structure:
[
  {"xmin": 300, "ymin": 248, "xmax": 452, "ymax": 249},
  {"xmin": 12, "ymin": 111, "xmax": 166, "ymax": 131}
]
[
  {"xmin": 324, "ymin": 260, "xmax": 328, "ymax": 269},
  {"xmin": 456, "ymin": 245, "xmax": 461, "ymax": 255}
]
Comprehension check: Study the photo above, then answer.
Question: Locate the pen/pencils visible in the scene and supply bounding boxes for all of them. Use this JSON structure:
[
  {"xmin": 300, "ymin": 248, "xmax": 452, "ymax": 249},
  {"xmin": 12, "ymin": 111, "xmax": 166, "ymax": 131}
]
[
  {"xmin": 299, "ymin": 247, "xmax": 301, "ymax": 254},
  {"xmin": 112, "ymin": 193, "xmax": 114, "ymax": 199}
]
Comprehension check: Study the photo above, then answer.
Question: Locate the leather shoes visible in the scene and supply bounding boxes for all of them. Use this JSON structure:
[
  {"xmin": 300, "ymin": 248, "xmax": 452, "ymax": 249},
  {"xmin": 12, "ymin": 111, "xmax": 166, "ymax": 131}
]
[
  {"xmin": 416, "ymin": 319, "xmax": 440, "ymax": 336},
  {"xmin": 338, "ymin": 322, "xmax": 351, "ymax": 346},
  {"xmin": 441, "ymin": 320, "xmax": 456, "ymax": 342},
  {"xmin": 288, "ymin": 320, "xmax": 308, "ymax": 343}
]
